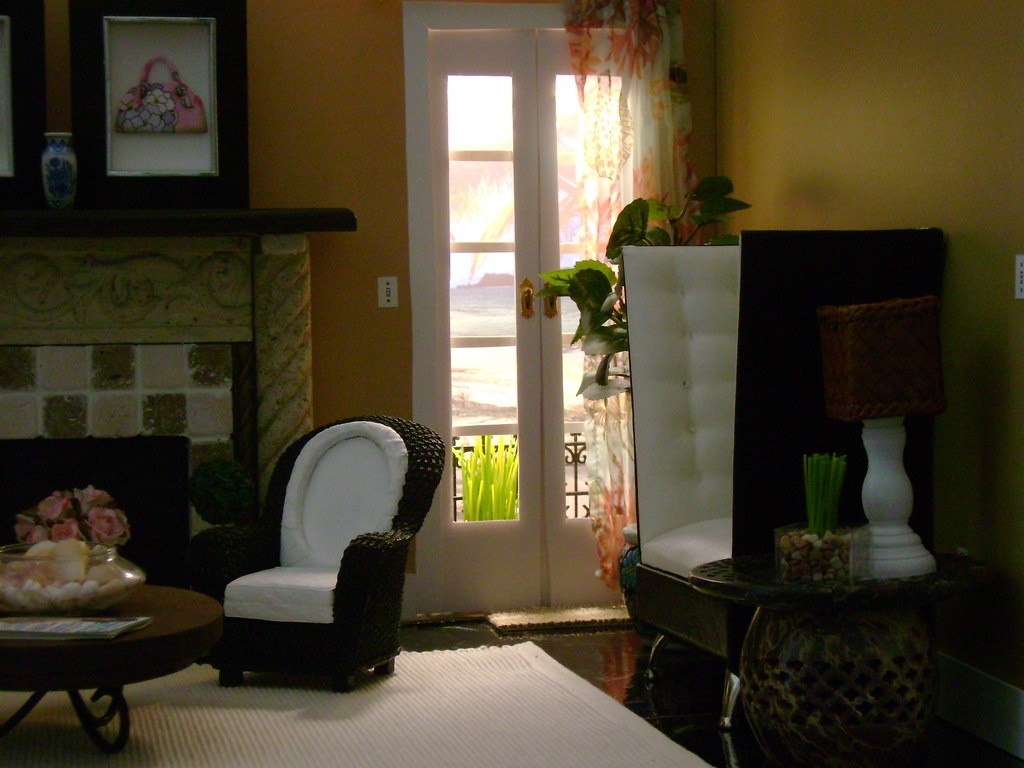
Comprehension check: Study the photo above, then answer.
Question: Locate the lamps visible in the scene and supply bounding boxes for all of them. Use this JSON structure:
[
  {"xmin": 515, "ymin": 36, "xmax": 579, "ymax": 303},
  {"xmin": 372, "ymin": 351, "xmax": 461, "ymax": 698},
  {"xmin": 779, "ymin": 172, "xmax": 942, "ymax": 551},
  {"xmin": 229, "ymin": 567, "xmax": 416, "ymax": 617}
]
[{"xmin": 819, "ymin": 292, "xmax": 947, "ymax": 581}]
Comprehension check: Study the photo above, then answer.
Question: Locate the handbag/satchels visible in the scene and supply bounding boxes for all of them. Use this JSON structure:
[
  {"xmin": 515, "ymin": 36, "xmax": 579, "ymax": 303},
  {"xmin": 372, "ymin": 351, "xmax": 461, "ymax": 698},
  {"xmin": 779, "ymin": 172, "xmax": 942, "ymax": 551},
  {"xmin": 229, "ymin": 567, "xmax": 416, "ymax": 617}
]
[{"xmin": 116, "ymin": 57, "xmax": 208, "ymax": 133}]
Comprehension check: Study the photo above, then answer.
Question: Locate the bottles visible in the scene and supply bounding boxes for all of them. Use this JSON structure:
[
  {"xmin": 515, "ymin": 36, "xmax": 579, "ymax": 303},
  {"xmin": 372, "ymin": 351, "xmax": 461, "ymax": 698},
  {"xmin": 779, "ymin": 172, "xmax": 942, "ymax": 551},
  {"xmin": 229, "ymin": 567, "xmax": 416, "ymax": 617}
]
[{"xmin": 40, "ymin": 132, "xmax": 77, "ymax": 211}]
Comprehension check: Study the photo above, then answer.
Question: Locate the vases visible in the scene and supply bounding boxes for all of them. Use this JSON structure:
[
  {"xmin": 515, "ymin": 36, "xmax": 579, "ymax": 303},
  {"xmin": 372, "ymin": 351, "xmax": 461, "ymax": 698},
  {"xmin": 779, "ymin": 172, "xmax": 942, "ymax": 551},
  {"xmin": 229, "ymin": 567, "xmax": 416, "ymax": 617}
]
[
  {"xmin": 41, "ymin": 130, "xmax": 80, "ymax": 210},
  {"xmin": 0, "ymin": 543, "xmax": 147, "ymax": 619}
]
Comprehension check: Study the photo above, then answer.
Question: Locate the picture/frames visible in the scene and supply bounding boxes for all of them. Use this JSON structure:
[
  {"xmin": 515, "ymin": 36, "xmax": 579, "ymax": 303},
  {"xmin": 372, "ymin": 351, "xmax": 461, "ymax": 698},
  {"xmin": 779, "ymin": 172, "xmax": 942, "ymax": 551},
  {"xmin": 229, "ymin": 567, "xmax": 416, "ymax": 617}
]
[
  {"xmin": 68, "ymin": 0, "xmax": 250, "ymax": 212},
  {"xmin": 0, "ymin": 0, "xmax": 49, "ymax": 208}
]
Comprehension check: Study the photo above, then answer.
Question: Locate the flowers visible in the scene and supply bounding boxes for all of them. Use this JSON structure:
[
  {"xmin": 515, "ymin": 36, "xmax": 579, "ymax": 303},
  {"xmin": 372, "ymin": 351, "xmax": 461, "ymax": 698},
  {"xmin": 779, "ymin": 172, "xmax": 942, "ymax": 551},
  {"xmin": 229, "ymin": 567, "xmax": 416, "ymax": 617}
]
[{"xmin": 13, "ymin": 484, "xmax": 133, "ymax": 559}]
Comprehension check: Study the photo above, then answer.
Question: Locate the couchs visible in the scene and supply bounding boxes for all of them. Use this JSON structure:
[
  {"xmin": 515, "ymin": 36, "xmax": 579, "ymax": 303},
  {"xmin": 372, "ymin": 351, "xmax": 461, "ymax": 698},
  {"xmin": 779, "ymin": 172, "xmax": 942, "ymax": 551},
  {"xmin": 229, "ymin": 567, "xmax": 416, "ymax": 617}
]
[{"xmin": 217, "ymin": 416, "xmax": 446, "ymax": 692}]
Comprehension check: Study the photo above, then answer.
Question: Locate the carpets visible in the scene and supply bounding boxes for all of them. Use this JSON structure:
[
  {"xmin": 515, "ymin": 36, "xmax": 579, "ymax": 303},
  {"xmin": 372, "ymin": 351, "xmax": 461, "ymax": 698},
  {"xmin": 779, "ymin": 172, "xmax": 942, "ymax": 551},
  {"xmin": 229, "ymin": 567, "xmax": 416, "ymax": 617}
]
[{"xmin": 0, "ymin": 644, "xmax": 719, "ymax": 768}]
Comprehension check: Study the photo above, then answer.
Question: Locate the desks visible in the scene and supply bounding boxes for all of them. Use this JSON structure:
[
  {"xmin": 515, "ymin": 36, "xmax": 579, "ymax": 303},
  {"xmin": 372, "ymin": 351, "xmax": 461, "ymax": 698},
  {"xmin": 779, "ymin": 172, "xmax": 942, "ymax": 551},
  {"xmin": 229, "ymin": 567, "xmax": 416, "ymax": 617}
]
[
  {"xmin": 0, "ymin": 585, "xmax": 223, "ymax": 753},
  {"xmin": 630, "ymin": 550, "xmax": 1023, "ymax": 768}
]
